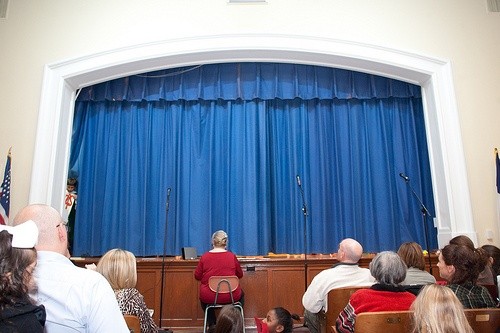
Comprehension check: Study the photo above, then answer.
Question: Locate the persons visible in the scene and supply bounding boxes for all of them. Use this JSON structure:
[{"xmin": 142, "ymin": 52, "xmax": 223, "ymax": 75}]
[
  {"xmin": 449, "ymin": 235, "xmax": 494, "ymax": 286},
  {"xmin": 406, "ymin": 282, "xmax": 476, "ymax": 333},
  {"xmin": 206, "ymin": 305, "xmax": 243, "ymax": 332},
  {"xmin": 397, "ymin": 241, "xmax": 437, "ymax": 286},
  {"xmin": 437, "ymin": 244, "xmax": 496, "ymax": 309},
  {"xmin": 14, "ymin": 204, "xmax": 130, "ymax": 333},
  {"xmin": 261, "ymin": 307, "xmax": 302, "ymax": 333},
  {"xmin": 194, "ymin": 229, "xmax": 244, "ymax": 328},
  {"xmin": 301, "ymin": 238, "xmax": 380, "ymax": 333},
  {"xmin": 0, "ymin": 225, "xmax": 46, "ymax": 333},
  {"xmin": 330, "ymin": 250, "xmax": 417, "ymax": 333},
  {"xmin": 63, "ymin": 178, "xmax": 78, "ymax": 227},
  {"xmin": 481, "ymin": 245, "xmax": 500, "ymax": 304},
  {"xmin": 95, "ymin": 248, "xmax": 160, "ymax": 333}
]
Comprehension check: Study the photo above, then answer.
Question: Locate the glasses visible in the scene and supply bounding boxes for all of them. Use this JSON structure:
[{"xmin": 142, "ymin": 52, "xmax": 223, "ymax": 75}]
[
  {"xmin": 56, "ymin": 219, "xmax": 68, "ymax": 232},
  {"xmin": 337, "ymin": 244, "xmax": 346, "ymax": 257}
]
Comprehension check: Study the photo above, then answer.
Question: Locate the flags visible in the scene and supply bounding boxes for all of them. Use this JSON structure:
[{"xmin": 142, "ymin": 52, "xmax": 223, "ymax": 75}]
[{"xmin": 0, "ymin": 154, "xmax": 11, "ymax": 225}]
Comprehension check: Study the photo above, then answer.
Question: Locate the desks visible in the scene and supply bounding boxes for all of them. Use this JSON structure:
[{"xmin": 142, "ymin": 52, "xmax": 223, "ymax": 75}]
[{"xmin": 68, "ymin": 252, "xmax": 450, "ymax": 328}]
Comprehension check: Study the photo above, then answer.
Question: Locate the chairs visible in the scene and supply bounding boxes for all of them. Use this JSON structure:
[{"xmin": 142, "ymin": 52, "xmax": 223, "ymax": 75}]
[
  {"xmin": 327, "ymin": 285, "xmax": 500, "ymax": 333},
  {"xmin": 202, "ymin": 276, "xmax": 246, "ymax": 333},
  {"xmin": 124, "ymin": 314, "xmax": 143, "ymax": 333}
]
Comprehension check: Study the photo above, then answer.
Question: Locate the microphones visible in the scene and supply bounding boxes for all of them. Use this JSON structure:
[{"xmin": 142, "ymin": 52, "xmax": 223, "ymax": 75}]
[
  {"xmin": 167, "ymin": 188, "xmax": 171, "ymax": 196},
  {"xmin": 399, "ymin": 173, "xmax": 409, "ymax": 179},
  {"xmin": 296, "ymin": 175, "xmax": 301, "ymax": 186}
]
